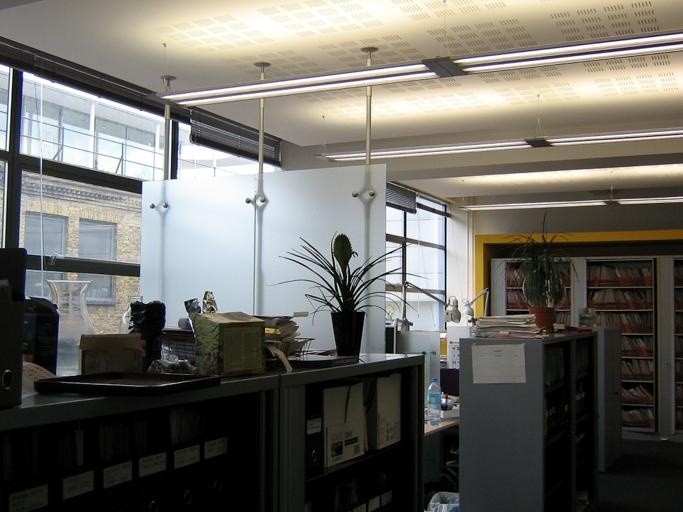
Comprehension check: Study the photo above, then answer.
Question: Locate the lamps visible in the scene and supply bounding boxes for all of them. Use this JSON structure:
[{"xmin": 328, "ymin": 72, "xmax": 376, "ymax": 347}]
[
  {"xmin": 462, "ymin": 288, "xmax": 492, "ymax": 318},
  {"xmin": 400, "ymin": 277, "xmax": 463, "ymax": 330}
]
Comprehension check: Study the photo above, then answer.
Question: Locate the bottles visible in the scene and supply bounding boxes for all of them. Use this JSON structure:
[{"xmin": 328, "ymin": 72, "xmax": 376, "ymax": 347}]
[
  {"xmin": 428, "ymin": 378, "xmax": 442, "ymax": 428},
  {"xmin": 440, "ymin": 333, "xmax": 448, "ymax": 362}
]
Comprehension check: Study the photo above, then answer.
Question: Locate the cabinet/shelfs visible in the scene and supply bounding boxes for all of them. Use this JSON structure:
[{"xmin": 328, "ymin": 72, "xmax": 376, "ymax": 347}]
[
  {"xmin": 457, "ymin": 324, "xmax": 600, "ymax": 512},
  {"xmin": 662, "ymin": 255, "xmax": 683, "ymax": 436},
  {"xmin": 0, "ymin": 366, "xmax": 277, "ymax": 512},
  {"xmin": 278, "ymin": 350, "xmax": 426, "ymax": 512},
  {"xmin": 492, "ymin": 255, "xmax": 661, "ymax": 443},
  {"xmin": 597, "ymin": 328, "xmax": 623, "ymax": 478}
]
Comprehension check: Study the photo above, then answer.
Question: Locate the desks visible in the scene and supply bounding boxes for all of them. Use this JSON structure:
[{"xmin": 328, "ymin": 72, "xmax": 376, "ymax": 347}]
[{"xmin": 423, "ymin": 391, "xmax": 457, "ymax": 495}]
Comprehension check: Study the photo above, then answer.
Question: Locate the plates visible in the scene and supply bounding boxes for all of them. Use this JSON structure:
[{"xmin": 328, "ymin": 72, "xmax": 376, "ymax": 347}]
[{"xmin": 33, "ymin": 370, "xmax": 221, "ymax": 397}]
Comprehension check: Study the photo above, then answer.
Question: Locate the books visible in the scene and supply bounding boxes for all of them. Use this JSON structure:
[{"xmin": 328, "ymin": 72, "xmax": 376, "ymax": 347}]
[
  {"xmin": 621, "ymin": 383, "xmax": 654, "ymax": 405},
  {"xmin": 588, "ymin": 290, "xmax": 653, "ymax": 309},
  {"xmin": 675, "ymin": 313, "xmax": 683, "ymax": 335},
  {"xmin": 554, "ymin": 313, "xmax": 569, "ymax": 326},
  {"xmin": 676, "ymin": 362, "xmax": 683, "ymax": 383},
  {"xmin": 620, "ymin": 409, "xmax": 653, "ymax": 429},
  {"xmin": 587, "ymin": 266, "xmax": 652, "ymax": 287},
  {"xmin": 556, "ymin": 264, "xmax": 572, "ymax": 287},
  {"xmin": 252, "ymin": 314, "xmax": 299, "ymax": 359},
  {"xmin": 554, "ymin": 289, "xmax": 570, "ymax": 310},
  {"xmin": 593, "ymin": 313, "xmax": 653, "ymax": 333},
  {"xmin": 507, "ymin": 290, "xmax": 528, "ymax": 309},
  {"xmin": 507, "ymin": 262, "xmax": 525, "ymax": 287},
  {"xmin": 675, "ymin": 338, "xmax": 682, "ymax": 359},
  {"xmin": 677, "ymin": 409, "xmax": 683, "ymax": 431},
  {"xmin": 674, "ymin": 289, "xmax": 682, "ymax": 311},
  {"xmin": 674, "ymin": 267, "xmax": 683, "ymax": 287},
  {"xmin": 675, "ymin": 387, "xmax": 683, "ymax": 407},
  {"xmin": 474, "ymin": 313, "xmax": 541, "ymax": 338},
  {"xmin": 621, "ymin": 359, "xmax": 653, "ymax": 381},
  {"xmin": 620, "ymin": 336, "xmax": 652, "ymax": 357},
  {"xmin": 163, "ymin": 328, "xmax": 196, "ymax": 364}
]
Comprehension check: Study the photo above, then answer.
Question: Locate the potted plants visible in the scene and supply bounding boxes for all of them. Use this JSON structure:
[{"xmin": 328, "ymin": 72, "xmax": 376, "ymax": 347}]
[
  {"xmin": 502, "ymin": 208, "xmax": 579, "ymax": 332},
  {"xmin": 277, "ymin": 231, "xmax": 423, "ymax": 356}
]
